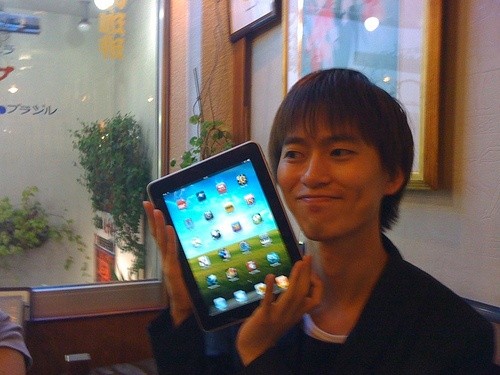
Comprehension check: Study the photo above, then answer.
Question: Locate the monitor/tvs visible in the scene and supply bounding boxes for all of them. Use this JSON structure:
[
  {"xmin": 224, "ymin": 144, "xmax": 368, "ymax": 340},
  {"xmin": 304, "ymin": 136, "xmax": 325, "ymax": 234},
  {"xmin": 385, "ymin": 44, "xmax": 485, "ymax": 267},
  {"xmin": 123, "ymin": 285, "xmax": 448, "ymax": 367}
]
[{"xmin": 226, "ymin": 0, "xmax": 282, "ymax": 43}]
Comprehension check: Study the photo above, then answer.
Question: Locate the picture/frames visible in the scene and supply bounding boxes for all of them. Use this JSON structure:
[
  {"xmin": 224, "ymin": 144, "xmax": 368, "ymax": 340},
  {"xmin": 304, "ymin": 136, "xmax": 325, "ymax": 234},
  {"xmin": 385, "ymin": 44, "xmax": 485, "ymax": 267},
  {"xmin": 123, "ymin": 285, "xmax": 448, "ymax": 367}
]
[{"xmin": 298, "ymin": 0, "xmax": 449, "ymax": 193}]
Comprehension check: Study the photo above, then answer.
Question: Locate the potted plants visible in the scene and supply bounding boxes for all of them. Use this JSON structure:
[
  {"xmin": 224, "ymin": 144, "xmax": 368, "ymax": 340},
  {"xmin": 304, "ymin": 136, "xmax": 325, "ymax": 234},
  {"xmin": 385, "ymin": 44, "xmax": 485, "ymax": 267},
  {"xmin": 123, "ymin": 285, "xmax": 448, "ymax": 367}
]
[{"xmin": 0, "ymin": 186, "xmax": 91, "ymax": 274}]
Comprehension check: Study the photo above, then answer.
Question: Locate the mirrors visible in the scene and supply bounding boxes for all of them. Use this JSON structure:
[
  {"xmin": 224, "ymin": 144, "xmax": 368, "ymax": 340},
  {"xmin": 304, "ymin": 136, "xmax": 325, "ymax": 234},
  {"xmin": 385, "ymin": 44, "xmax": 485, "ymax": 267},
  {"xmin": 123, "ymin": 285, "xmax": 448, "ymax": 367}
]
[{"xmin": 0, "ymin": 1, "xmax": 172, "ymax": 322}]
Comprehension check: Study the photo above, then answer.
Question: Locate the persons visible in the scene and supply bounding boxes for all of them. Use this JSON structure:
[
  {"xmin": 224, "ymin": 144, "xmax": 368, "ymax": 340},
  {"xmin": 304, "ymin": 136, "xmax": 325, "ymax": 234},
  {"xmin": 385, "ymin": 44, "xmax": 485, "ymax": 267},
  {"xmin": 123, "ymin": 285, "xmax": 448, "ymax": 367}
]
[
  {"xmin": 0, "ymin": 307, "xmax": 35, "ymax": 375},
  {"xmin": 142, "ymin": 69, "xmax": 500, "ymax": 375}
]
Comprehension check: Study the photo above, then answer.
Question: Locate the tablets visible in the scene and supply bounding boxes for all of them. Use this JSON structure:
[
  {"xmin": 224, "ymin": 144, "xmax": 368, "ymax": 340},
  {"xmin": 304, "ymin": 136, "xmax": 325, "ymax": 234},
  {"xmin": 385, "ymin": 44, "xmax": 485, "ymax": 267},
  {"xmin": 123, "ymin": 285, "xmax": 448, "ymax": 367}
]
[{"xmin": 145, "ymin": 140, "xmax": 311, "ymax": 337}]
